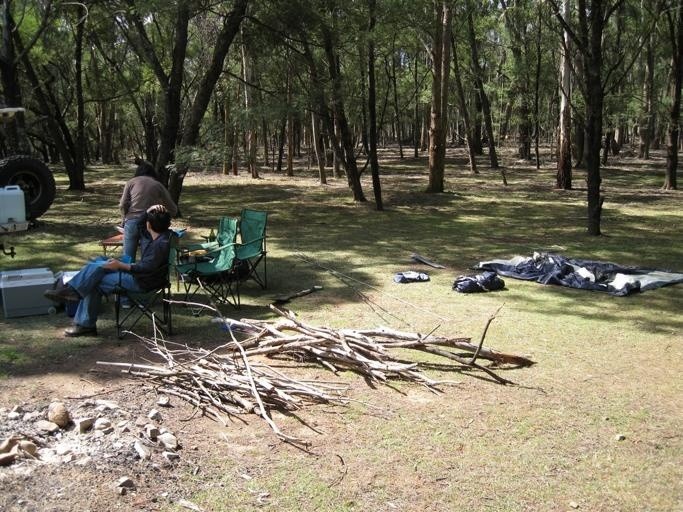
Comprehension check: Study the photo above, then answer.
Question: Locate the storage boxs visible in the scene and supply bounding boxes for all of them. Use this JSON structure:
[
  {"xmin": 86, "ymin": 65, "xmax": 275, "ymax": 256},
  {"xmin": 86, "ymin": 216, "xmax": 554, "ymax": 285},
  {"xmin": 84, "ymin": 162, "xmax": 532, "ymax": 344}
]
[{"xmin": 0, "ymin": 267, "xmax": 64, "ymax": 318}]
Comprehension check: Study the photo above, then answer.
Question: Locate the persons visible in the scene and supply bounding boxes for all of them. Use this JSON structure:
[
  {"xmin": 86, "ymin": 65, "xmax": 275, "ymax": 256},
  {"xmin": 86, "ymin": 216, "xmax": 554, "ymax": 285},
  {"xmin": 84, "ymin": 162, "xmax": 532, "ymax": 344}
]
[
  {"xmin": 41, "ymin": 204, "xmax": 172, "ymax": 336},
  {"xmin": 114, "ymin": 160, "xmax": 178, "ymax": 311}
]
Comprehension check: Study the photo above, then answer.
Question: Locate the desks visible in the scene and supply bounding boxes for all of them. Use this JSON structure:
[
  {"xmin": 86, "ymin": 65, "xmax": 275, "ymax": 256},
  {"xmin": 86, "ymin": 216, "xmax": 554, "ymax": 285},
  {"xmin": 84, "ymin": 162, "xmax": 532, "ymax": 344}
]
[{"xmin": 99, "ymin": 234, "xmax": 179, "ymax": 265}]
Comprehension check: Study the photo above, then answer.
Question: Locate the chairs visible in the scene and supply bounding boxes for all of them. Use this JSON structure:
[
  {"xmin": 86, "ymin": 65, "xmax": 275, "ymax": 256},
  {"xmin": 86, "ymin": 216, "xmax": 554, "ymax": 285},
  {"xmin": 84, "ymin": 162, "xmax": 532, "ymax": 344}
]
[
  {"xmin": 173, "ymin": 208, "xmax": 269, "ymax": 317},
  {"xmin": 110, "ymin": 230, "xmax": 175, "ymax": 341}
]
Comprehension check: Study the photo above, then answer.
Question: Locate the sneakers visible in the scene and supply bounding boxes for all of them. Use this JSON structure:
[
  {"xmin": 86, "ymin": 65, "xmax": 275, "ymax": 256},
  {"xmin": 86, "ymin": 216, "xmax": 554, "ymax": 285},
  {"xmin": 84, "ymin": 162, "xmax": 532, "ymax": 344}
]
[
  {"xmin": 43, "ymin": 286, "xmax": 80, "ymax": 305},
  {"xmin": 65, "ymin": 326, "xmax": 97, "ymax": 336}
]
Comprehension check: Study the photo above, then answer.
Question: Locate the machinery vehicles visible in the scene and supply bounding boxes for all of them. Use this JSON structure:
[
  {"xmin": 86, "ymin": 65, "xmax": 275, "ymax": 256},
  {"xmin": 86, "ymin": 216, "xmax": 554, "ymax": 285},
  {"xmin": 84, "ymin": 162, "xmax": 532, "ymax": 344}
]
[{"xmin": 0, "ymin": 103, "xmax": 59, "ymax": 223}]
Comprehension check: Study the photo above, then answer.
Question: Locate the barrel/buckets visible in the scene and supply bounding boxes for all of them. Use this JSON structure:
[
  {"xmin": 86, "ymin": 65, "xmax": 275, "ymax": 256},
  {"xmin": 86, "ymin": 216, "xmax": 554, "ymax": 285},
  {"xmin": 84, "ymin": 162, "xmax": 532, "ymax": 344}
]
[{"xmin": 0, "ymin": 185, "xmax": 28, "ymax": 224}]
